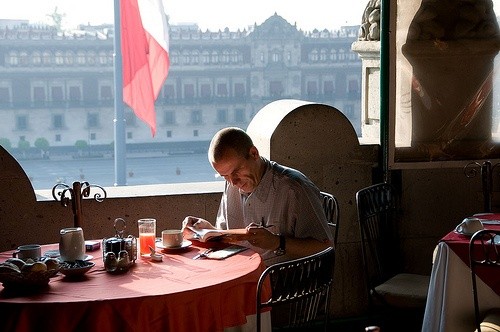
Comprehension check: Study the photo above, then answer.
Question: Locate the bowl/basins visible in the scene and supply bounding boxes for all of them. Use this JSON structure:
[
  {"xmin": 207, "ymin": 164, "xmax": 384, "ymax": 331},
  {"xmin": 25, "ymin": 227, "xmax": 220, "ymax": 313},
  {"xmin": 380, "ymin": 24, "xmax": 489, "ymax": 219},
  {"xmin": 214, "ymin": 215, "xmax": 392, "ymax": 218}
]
[{"xmin": 60, "ymin": 259, "xmax": 94, "ymax": 278}]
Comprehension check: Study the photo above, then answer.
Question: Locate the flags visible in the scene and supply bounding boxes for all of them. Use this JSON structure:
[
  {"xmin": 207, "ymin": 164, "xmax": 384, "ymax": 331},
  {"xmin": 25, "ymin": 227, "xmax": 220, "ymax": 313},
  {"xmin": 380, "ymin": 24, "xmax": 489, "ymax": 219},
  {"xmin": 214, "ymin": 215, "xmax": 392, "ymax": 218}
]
[{"xmin": 119, "ymin": 0, "xmax": 172, "ymax": 137}]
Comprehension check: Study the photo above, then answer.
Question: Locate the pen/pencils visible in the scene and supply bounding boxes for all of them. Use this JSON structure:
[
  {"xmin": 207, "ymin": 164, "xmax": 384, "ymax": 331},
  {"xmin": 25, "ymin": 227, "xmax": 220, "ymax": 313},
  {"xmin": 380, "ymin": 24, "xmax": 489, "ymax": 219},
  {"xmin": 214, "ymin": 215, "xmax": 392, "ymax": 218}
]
[{"xmin": 261, "ymin": 217, "xmax": 264, "ymax": 226}]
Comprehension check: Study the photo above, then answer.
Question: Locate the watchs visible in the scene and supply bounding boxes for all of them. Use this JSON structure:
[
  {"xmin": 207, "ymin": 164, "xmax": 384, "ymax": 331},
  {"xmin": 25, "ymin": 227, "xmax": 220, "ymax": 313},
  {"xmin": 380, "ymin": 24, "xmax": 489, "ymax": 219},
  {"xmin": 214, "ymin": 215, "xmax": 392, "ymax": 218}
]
[{"xmin": 274, "ymin": 235, "xmax": 286, "ymax": 257}]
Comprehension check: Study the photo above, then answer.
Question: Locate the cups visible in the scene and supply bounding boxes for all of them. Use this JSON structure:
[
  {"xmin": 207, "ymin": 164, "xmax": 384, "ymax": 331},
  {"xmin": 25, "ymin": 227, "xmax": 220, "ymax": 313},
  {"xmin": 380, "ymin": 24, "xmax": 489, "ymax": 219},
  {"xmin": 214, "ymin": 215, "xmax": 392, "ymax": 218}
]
[
  {"xmin": 162, "ymin": 230, "xmax": 183, "ymax": 247},
  {"xmin": 456, "ymin": 217, "xmax": 484, "ymax": 235},
  {"xmin": 60, "ymin": 227, "xmax": 85, "ymax": 261},
  {"xmin": 137, "ymin": 218, "xmax": 156, "ymax": 258},
  {"xmin": 13, "ymin": 245, "xmax": 41, "ymax": 260}
]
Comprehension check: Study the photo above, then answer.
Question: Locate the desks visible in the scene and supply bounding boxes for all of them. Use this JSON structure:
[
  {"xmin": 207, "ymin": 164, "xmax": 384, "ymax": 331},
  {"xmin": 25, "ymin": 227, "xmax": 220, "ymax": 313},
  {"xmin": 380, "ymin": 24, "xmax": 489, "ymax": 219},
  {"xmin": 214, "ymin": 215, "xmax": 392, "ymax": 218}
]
[
  {"xmin": 0, "ymin": 232, "xmax": 272, "ymax": 332},
  {"xmin": 421, "ymin": 213, "xmax": 500, "ymax": 332}
]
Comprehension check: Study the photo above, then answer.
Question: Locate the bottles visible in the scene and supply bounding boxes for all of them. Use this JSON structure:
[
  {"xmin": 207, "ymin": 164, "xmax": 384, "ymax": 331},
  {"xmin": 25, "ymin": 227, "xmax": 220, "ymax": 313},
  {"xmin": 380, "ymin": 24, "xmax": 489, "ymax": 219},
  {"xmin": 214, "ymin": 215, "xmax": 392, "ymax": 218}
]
[
  {"xmin": 117, "ymin": 250, "xmax": 129, "ymax": 271},
  {"xmin": 105, "ymin": 253, "xmax": 117, "ymax": 272}
]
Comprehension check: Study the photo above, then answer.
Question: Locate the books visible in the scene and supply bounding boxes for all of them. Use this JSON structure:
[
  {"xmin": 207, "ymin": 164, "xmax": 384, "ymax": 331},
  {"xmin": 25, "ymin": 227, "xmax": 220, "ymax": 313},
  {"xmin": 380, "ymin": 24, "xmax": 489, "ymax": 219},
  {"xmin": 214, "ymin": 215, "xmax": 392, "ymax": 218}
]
[{"xmin": 185, "ymin": 225, "xmax": 249, "ymax": 243}]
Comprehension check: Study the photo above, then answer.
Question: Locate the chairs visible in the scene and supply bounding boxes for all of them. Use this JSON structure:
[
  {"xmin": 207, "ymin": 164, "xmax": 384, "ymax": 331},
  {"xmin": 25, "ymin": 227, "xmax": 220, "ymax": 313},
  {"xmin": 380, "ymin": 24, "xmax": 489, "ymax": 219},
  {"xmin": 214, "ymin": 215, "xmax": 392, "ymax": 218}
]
[
  {"xmin": 480, "ymin": 161, "xmax": 500, "ymax": 217},
  {"xmin": 256, "ymin": 245, "xmax": 336, "ymax": 332},
  {"xmin": 355, "ymin": 181, "xmax": 431, "ymax": 327},
  {"xmin": 468, "ymin": 229, "xmax": 500, "ymax": 332},
  {"xmin": 319, "ymin": 191, "xmax": 340, "ymax": 248}
]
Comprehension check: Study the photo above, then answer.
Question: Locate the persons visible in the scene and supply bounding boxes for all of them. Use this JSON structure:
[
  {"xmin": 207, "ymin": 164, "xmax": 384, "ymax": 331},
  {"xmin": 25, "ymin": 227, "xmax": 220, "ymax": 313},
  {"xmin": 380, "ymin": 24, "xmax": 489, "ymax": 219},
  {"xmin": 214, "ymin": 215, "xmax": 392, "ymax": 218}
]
[{"xmin": 181, "ymin": 125, "xmax": 334, "ymax": 300}]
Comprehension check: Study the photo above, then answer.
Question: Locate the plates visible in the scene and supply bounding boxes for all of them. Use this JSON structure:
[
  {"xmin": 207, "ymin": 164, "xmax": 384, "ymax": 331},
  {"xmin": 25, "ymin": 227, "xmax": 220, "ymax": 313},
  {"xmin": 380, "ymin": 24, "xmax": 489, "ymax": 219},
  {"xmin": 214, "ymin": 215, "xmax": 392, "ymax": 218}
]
[
  {"xmin": 155, "ymin": 239, "xmax": 192, "ymax": 250},
  {"xmin": 43, "ymin": 249, "xmax": 60, "ymax": 257},
  {"xmin": 57, "ymin": 254, "xmax": 94, "ymax": 262}
]
